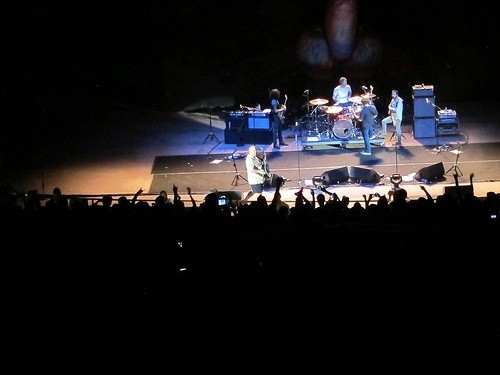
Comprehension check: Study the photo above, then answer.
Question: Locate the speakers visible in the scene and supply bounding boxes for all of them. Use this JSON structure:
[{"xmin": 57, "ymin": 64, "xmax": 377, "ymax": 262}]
[
  {"xmin": 265, "ymin": 173, "xmax": 282, "ymax": 187},
  {"xmin": 320, "ymin": 166, "xmax": 380, "ymax": 185},
  {"xmin": 415, "ymin": 162, "xmax": 445, "ymax": 182},
  {"xmin": 413, "ymin": 96, "xmax": 437, "ymax": 138}
]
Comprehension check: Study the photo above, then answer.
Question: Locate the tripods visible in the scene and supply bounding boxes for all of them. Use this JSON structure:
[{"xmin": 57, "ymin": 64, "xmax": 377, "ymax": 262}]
[
  {"xmin": 231, "ymin": 150, "xmax": 248, "ymax": 185},
  {"xmin": 203, "ymin": 108, "xmax": 219, "ymax": 144},
  {"xmin": 446, "ymin": 141, "xmax": 464, "ymax": 175}
]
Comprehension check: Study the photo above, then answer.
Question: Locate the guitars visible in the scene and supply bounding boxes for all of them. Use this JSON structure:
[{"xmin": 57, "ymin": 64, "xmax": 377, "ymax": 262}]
[{"xmin": 278, "ymin": 95, "xmax": 288, "ymax": 122}]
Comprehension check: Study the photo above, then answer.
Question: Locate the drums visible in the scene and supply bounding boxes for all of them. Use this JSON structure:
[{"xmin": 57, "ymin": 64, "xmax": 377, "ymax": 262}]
[{"xmin": 332, "ymin": 115, "xmax": 354, "ymax": 138}]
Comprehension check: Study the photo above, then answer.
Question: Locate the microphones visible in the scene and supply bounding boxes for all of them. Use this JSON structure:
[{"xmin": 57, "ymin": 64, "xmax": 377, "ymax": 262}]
[
  {"xmin": 425, "ymin": 98, "xmax": 429, "ymax": 103},
  {"xmin": 370, "ymin": 85, "xmax": 373, "ymax": 90},
  {"xmin": 361, "ymin": 86, "xmax": 367, "ymax": 90},
  {"xmin": 304, "ymin": 90, "xmax": 309, "ymax": 93}
]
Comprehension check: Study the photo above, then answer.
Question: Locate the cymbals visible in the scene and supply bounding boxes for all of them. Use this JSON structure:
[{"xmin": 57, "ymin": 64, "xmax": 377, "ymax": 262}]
[
  {"xmin": 325, "ymin": 106, "xmax": 343, "ymax": 113},
  {"xmin": 309, "ymin": 98, "xmax": 329, "ymax": 105},
  {"xmin": 360, "ymin": 93, "xmax": 376, "ymax": 98},
  {"xmin": 347, "ymin": 97, "xmax": 363, "ymax": 103}
]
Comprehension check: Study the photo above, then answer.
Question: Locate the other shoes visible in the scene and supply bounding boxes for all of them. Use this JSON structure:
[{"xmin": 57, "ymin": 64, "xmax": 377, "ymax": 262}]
[
  {"xmin": 361, "ymin": 152, "xmax": 371, "ymax": 155},
  {"xmin": 279, "ymin": 143, "xmax": 288, "ymax": 145},
  {"xmin": 274, "ymin": 145, "xmax": 280, "ymax": 149}
]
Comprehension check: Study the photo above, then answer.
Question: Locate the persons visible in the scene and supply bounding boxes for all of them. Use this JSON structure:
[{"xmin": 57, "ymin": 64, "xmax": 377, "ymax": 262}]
[
  {"xmin": 246, "ymin": 146, "xmax": 268, "ymax": 193},
  {"xmin": 269, "ymin": 89, "xmax": 289, "ymax": 149},
  {"xmin": 332, "ymin": 77, "xmax": 351, "ymax": 107},
  {"xmin": 382, "ymin": 89, "xmax": 403, "ymax": 144},
  {"xmin": 354, "ymin": 97, "xmax": 379, "ymax": 154},
  {"xmin": 21, "ymin": 173, "xmax": 479, "ymax": 219}
]
